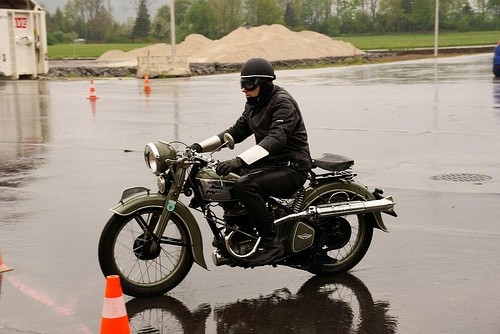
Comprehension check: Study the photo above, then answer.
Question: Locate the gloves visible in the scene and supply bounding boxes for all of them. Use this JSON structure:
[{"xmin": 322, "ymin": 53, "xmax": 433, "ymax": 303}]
[
  {"xmin": 185, "ymin": 143, "xmax": 203, "ymax": 157},
  {"xmin": 215, "ymin": 158, "xmax": 244, "ymax": 176}
]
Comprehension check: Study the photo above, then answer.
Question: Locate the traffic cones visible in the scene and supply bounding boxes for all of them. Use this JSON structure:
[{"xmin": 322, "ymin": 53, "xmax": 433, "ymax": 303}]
[
  {"xmin": 144, "ymin": 75, "xmax": 151, "ymax": 91},
  {"xmin": 99, "ymin": 275, "xmax": 132, "ymax": 334},
  {"xmin": 86, "ymin": 80, "xmax": 99, "ymax": 99}
]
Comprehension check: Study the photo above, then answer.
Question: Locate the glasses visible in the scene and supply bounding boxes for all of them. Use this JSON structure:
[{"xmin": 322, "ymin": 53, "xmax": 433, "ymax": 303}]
[{"xmin": 240, "ymin": 77, "xmax": 269, "ymax": 92}]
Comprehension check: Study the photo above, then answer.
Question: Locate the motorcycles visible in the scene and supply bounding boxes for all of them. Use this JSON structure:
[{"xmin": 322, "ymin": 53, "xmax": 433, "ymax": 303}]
[{"xmin": 97, "ymin": 133, "xmax": 398, "ymax": 300}]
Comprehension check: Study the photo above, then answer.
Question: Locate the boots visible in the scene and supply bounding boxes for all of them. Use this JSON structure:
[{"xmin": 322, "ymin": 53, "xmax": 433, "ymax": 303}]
[{"xmin": 244, "ymin": 206, "xmax": 287, "ymax": 265}]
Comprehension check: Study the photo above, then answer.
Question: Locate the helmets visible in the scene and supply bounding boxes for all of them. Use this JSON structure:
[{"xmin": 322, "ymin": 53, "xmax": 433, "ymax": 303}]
[{"xmin": 241, "ymin": 57, "xmax": 276, "ymax": 80}]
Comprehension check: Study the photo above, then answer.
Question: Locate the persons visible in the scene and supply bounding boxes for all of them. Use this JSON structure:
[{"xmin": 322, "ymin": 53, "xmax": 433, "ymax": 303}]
[{"xmin": 186, "ymin": 58, "xmax": 313, "ymax": 263}]
